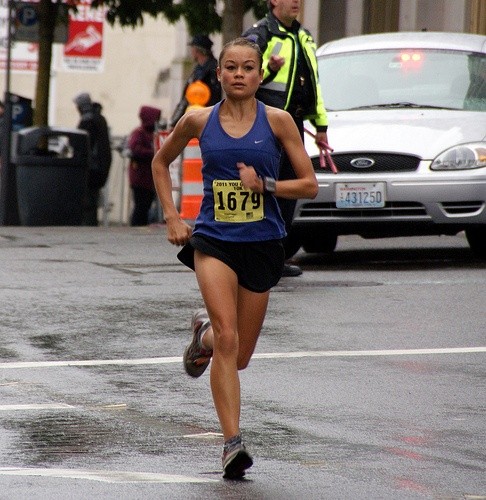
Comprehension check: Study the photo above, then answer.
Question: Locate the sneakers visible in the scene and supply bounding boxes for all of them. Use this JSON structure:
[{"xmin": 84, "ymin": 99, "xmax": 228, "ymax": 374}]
[
  {"xmin": 183, "ymin": 308, "xmax": 213, "ymax": 377},
  {"xmin": 222, "ymin": 437, "xmax": 253, "ymax": 479},
  {"xmin": 281, "ymin": 263, "xmax": 302, "ymax": 276}
]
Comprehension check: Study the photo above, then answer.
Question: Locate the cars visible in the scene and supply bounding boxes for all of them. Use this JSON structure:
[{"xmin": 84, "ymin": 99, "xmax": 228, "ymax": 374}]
[{"xmin": 289, "ymin": 30, "xmax": 486, "ymax": 254}]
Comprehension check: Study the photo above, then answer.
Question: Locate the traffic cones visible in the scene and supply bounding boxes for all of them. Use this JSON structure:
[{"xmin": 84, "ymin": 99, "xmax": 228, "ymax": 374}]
[{"xmin": 179, "ymin": 137, "xmax": 204, "ymax": 226}]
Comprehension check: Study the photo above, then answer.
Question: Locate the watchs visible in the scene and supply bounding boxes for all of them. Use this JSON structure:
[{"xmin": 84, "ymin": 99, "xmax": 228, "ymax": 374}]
[{"xmin": 263, "ymin": 175, "xmax": 277, "ymax": 194}]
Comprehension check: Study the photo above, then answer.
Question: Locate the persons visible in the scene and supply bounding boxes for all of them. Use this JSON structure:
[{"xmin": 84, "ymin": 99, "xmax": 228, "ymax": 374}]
[
  {"xmin": 151, "ymin": 38, "xmax": 319, "ymax": 480},
  {"xmin": 72, "ymin": 92, "xmax": 112, "ymax": 226},
  {"xmin": 169, "ymin": 34, "xmax": 222, "ymax": 129},
  {"xmin": 127, "ymin": 106, "xmax": 161, "ymax": 226},
  {"xmin": 236, "ymin": 0, "xmax": 328, "ymax": 278}
]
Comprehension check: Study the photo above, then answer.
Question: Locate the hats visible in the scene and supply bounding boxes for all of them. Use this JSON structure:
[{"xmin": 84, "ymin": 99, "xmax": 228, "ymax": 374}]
[
  {"xmin": 187, "ymin": 36, "xmax": 213, "ymax": 48},
  {"xmin": 73, "ymin": 93, "xmax": 91, "ymax": 104}
]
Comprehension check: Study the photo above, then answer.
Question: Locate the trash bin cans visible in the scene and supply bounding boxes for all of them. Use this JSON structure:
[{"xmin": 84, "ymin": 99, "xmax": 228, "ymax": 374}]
[{"xmin": 10, "ymin": 126, "xmax": 90, "ymax": 225}]
